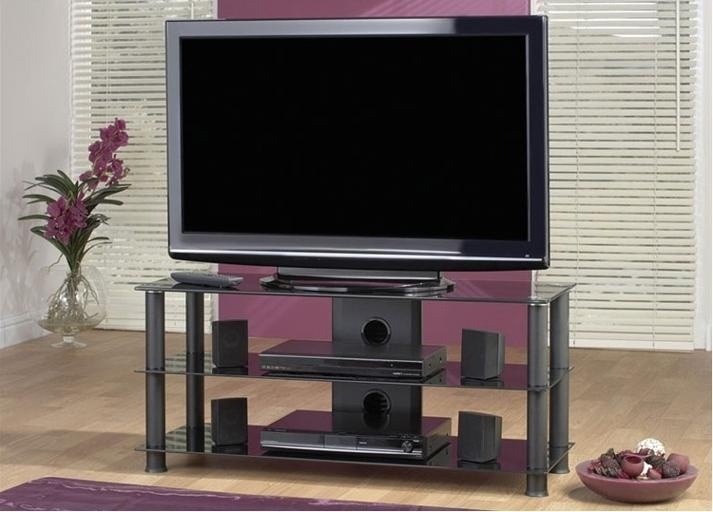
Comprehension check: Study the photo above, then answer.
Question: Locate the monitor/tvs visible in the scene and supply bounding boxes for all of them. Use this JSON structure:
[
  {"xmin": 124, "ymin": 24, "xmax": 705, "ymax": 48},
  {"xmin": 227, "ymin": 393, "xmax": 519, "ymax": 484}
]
[{"xmin": 163, "ymin": 14, "xmax": 551, "ymax": 296}]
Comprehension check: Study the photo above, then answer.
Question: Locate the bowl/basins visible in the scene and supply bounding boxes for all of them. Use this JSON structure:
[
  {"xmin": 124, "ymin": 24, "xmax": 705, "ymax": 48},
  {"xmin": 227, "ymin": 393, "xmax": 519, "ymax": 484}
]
[{"xmin": 576, "ymin": 459, "xmax": 699, "ymax": 504}]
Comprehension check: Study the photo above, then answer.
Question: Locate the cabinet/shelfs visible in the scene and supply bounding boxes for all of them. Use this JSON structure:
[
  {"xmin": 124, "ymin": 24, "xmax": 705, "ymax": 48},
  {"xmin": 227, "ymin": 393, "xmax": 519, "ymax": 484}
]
[{"xmin": 134, "ymin": 271, "xmax": 577, "ymax": 497}]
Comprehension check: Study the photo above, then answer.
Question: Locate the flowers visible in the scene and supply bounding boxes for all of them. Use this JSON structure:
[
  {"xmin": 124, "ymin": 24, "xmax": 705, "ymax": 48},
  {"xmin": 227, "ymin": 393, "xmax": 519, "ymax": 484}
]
[{"xmin": 16, "ymin": 117, "xmax": 133, "ymax": 333}]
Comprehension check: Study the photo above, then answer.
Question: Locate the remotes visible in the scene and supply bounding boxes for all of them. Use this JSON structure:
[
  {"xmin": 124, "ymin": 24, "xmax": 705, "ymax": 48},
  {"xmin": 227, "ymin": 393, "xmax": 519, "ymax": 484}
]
[{"xmin": 171, "ymin": 270, "xmax": 244, "ymax": 288}]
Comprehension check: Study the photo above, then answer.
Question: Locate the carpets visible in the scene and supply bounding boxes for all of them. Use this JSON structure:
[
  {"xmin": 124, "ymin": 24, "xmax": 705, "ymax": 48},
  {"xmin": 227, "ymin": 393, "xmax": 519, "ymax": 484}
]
[{"xmin": 0, "ymin": 476, "xmax": 491, "ymax": 510}]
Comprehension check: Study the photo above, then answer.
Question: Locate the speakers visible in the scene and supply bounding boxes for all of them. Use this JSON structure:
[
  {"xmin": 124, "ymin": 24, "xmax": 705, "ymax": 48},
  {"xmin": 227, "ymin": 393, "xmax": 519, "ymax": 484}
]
[
  {"xmin": 457, "ymin": 411, "xmax": 503, "ymax": 463},
  {"xmin": 461, "ymin": 328, "xmax": 505, "ymax": 379},
  {"xmin": 212, "ymin": 319, "xmax": 249, "ymax": 369},
  {"xmin": 211, "ymin": 397, "xmax": 248, "ymax": 447}
]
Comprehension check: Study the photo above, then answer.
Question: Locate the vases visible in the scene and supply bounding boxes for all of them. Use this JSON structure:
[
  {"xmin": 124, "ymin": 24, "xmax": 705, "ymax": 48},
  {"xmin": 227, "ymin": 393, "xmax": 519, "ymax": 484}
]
[{"xmin": 25, "ymin": 265, "xmax": 107, "ymax": 349}]
